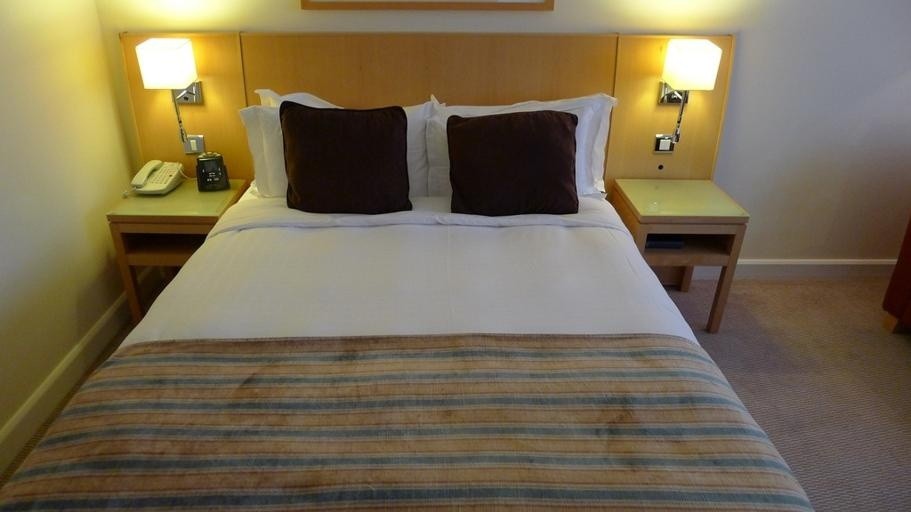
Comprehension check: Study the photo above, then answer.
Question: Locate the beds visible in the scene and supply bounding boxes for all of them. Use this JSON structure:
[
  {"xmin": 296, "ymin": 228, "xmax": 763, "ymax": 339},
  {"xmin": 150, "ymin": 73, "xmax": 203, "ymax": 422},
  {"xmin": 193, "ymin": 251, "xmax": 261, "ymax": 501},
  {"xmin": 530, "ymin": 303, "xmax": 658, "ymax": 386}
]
[{"xmin": 0, "ymin": 31, "xmax": 814, "ymax": 512}]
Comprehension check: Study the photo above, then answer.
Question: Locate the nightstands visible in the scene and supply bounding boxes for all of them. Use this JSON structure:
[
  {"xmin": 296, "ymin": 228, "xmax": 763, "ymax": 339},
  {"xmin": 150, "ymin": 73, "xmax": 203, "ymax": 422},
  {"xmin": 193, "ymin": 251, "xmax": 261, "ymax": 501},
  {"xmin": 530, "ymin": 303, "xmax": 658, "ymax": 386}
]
[
  {"xmin": 611, "ymin": 178, "xmax": 751, "ymax": 335},
  {"xmin": 106, "ymin": 178, "xmax": 248, "ymax": 328}
]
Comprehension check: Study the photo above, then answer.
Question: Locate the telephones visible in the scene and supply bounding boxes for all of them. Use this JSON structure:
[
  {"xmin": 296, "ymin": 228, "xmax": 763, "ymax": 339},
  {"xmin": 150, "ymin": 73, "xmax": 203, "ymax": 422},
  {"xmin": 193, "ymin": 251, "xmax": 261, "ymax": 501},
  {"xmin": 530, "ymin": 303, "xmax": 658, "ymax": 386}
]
[{"xmin": 122, "ymin": 160, "xmax": 184, "ymax": 198}]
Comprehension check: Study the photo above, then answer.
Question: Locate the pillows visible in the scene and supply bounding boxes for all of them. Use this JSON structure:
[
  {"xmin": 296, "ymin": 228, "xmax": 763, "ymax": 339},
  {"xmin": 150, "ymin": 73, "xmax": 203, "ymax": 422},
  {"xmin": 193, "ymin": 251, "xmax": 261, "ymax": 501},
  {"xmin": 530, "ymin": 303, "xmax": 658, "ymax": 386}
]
[
  {"xmin": 240, "ymin": 105, "xmax": 288, "ymax": 199},
  {"xmin": 279, "ymin": 100, "xmax": 415, "ymax": 215},
  {"xmin": 446, "ymin": 111, "xmax": 580, "ymax": 216},
  {"xmin": 254, "ymin": 89, "xmax": 432, "ymax": 198},
  {"xmin": 427, "ymin": 93, "xmax": 617, "ymax": 197}
]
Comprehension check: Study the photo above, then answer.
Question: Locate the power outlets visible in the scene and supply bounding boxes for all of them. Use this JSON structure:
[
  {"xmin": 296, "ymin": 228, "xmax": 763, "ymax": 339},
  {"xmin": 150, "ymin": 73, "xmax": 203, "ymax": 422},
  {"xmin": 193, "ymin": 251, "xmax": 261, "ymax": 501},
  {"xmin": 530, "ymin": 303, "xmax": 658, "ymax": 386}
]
[
  {"xmin": 184, "ymin": 134, "xmax": 205, "ymax": 155},
  {"xmin": 653, "ymin": 134, "xmax": 675, "ymax": 151}
]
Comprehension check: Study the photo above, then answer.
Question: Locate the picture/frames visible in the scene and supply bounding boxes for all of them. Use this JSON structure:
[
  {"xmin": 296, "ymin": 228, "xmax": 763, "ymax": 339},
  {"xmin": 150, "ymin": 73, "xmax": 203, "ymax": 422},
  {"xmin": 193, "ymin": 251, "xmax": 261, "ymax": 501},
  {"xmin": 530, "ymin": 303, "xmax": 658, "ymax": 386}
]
[{"xmin": 300, "ymin": 0, "xmax": 554, "ymax": 11}]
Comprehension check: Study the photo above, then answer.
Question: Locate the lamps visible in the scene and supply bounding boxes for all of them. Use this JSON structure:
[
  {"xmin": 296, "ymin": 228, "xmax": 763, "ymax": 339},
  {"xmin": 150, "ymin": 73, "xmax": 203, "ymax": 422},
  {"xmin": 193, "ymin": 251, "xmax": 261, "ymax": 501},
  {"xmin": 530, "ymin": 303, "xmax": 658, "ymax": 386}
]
[
  {"xmin": 662, "ymin": 39, "xmax": 722, "ymax": 91},
  {"xmin": 135, "ymin": 37, "xmax": 198, "ymax": 88}
]
